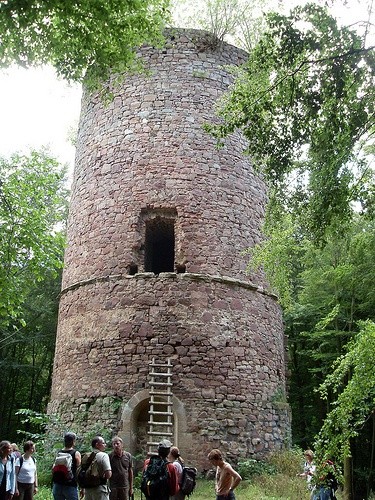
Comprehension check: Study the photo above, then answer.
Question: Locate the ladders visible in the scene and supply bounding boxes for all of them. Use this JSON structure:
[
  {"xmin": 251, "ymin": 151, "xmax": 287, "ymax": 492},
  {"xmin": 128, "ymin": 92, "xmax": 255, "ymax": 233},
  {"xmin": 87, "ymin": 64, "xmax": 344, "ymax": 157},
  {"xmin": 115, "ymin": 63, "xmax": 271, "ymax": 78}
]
[{"xmin": 145, "ymin": 357, "xmax": 173, "ymax": 461}]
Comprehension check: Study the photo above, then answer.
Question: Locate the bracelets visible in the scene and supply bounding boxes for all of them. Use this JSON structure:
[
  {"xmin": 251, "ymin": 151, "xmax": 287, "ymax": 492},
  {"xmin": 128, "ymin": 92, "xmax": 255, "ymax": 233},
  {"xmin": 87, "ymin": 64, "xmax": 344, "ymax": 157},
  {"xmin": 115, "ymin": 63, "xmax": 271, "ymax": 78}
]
[
  {"xmin": 229, "ymin": 487, "xmax": 234, "ymax": 492},
  {"xmin": 35, "ymin": 486, "xmax": 38, "ymax": 488},
  {"xmin": 130, "ymin": 488, "xmax": 133, "ymax": 489}
]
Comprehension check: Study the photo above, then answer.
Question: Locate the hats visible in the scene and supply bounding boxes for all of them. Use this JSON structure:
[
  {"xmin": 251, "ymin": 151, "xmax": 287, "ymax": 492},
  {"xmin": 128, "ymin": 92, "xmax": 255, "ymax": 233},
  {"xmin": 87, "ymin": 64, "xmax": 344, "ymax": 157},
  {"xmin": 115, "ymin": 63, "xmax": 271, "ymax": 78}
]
[
  {"xmin": 170, "ymin": 446, "xmax": 179, "ymax": 455},
  {"xmin": 159, "ymin": 440, "xmax": 173, "ymax": 448}
]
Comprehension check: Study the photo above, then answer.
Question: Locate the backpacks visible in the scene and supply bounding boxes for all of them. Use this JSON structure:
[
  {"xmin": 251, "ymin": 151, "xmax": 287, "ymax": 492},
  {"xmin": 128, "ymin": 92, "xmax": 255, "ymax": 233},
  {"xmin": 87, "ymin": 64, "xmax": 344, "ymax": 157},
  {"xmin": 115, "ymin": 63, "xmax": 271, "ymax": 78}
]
[
  {"xmin": 141, "ymin": 455, "xmax": 173, "ymax": 500},
  {"xmin": 77, "ymin": 450, "xmax": 107, "ymax": 488},
  {"xmin": 175, "ymin": 460, "xmax": 197, "ymax": 495},
  {"xmin": 51, "ymin": 449, "xmax": 77, "ymax": 487}
]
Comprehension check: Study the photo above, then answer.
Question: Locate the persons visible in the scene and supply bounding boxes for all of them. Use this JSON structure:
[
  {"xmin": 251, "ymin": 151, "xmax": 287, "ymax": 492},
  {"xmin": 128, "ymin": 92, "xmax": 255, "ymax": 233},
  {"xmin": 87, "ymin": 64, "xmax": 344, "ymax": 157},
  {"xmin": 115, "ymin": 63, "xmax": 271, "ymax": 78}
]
[
  {"xmin": 81, "ymin": 436, "xmax": 112, "ymax": 500},
  {"xmin": 296, "ymin": 449, "xmax": 339, "ymax": 500},
  {"xmin": 15, "ymin": 440, "xmax": 39, "ymax": 500},
  {"xmin": 207, "ymin": 449, "xmax": 242, "ymax": 500},
  {"xmin": 51, "ymin": 432, "xmax": 84, "ymax": 500},
  {"xmin": 0, "ymin": 440, "xmax": 15, "ymax": 500},
  {"xmin": 140, "ymin": 439, "xmax": 178, "ymax": 500},
  {"xmin": 168, "ymin": 446, "xmax": 198, "ymax": 500},
  {"xmin": 11, "ymin": 442, "xmax": 21, "ymax": 460},
  {"xmin": 107, "ymin": 436, "xmax": 134, "ymax": 500}
]
